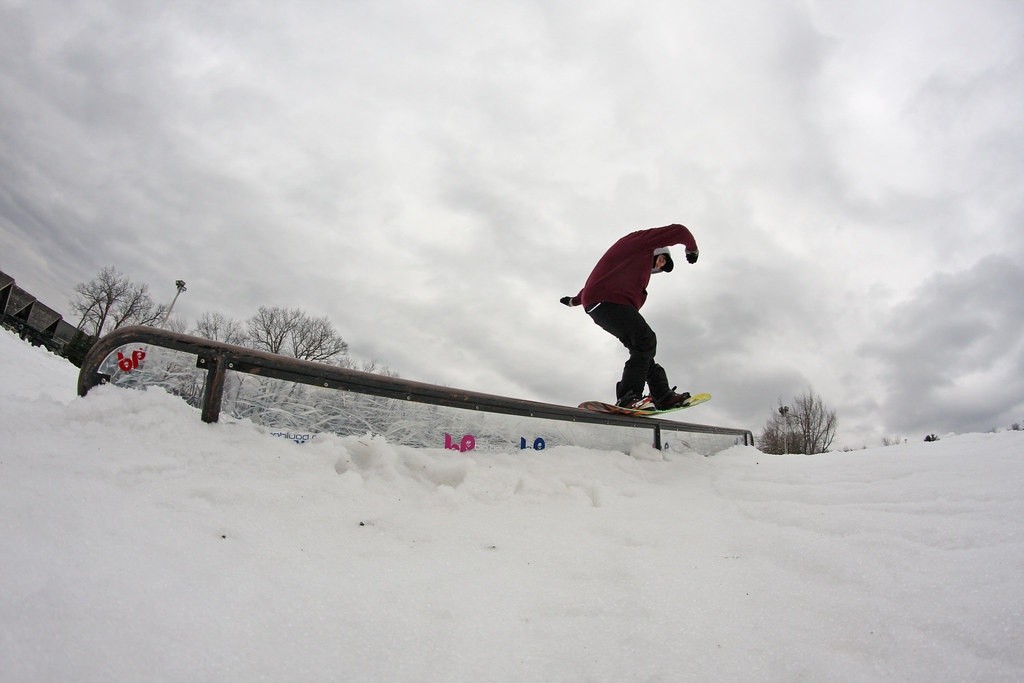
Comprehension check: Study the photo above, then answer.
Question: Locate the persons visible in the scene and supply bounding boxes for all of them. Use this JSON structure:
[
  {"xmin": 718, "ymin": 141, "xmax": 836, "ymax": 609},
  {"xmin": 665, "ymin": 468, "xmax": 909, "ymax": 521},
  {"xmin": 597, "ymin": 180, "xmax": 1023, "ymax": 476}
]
[{"xmin": 559, "ymin": 223, "xmax": 698, "ymax": 411}]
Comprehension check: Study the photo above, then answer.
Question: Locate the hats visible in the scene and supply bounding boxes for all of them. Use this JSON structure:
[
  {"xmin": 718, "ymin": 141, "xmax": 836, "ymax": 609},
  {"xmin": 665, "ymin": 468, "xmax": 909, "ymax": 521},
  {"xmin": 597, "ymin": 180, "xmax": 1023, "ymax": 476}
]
[{"xmin": 661, "ymin": 252, "xmax": 673, "ymax": 273}]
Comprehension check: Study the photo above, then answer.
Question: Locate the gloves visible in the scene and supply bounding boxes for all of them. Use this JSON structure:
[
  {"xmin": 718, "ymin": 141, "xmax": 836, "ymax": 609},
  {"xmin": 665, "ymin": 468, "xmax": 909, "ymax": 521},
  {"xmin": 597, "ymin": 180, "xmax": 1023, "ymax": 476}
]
[
  {"xmin": 559, "ymin": 296, "xmax": 573, "ymax": 307},
  {"xmin": 685, "ymin": 247, "xmax": 700, "ymax": 264}
]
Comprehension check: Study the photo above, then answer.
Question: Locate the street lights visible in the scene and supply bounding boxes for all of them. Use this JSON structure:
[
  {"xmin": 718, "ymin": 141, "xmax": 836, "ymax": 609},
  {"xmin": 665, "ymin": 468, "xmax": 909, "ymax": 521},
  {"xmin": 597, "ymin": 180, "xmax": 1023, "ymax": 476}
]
[{"xmin": 157, "ymin": 279, "xmax": 188, "ymax": 329}]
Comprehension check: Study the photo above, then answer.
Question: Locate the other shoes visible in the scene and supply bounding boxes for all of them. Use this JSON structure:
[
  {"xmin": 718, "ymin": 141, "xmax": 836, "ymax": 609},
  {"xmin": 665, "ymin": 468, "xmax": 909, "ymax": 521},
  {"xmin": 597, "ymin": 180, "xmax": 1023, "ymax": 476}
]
[
  {"xmin": 649, "ymin": 390, "xmax": 691, "ymax": 410},
  {"xmin": 615, "ymin": 390, "xmax": 655, "ymax": 410}
]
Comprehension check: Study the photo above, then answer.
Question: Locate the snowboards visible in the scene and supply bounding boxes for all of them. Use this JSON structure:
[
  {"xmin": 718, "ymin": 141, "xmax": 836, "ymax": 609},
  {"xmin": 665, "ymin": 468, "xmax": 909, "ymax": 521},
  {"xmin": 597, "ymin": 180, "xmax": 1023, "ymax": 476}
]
[{"xmin": 577, "ymin": 392, "xmax": 712, "ymax": 416}]
